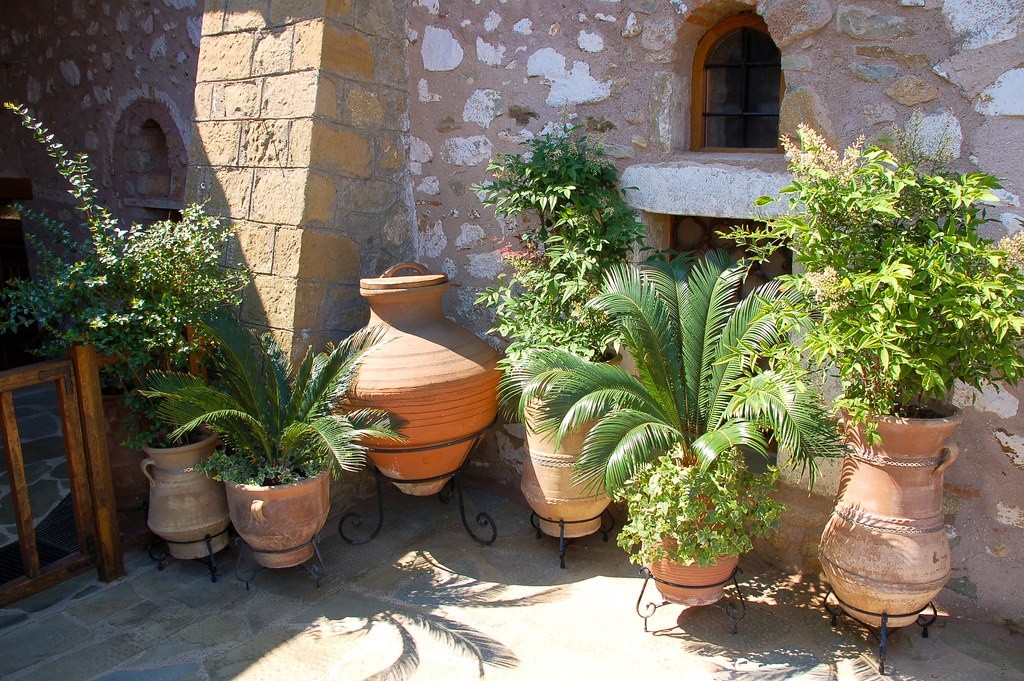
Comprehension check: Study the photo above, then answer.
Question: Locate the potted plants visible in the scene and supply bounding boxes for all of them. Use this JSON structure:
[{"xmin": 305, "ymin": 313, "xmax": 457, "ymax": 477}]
[
  {"xmin": 0, "ymin": 100, "xmax": 256, "ymax": 560},
  {"xmin": 495, "ymin": 246, "xmax": 853, "ymax": 607},
  {"xmin": 715, "ymin": 105, "xmax": 1024, "ymax": 629},
  {"xmin": 118, "ymin": 307, "xmax": 411, "ymax": 569},
  {"xmin": 469, "ymin": 98, "xmax": 678, "ymax": 539}
]
